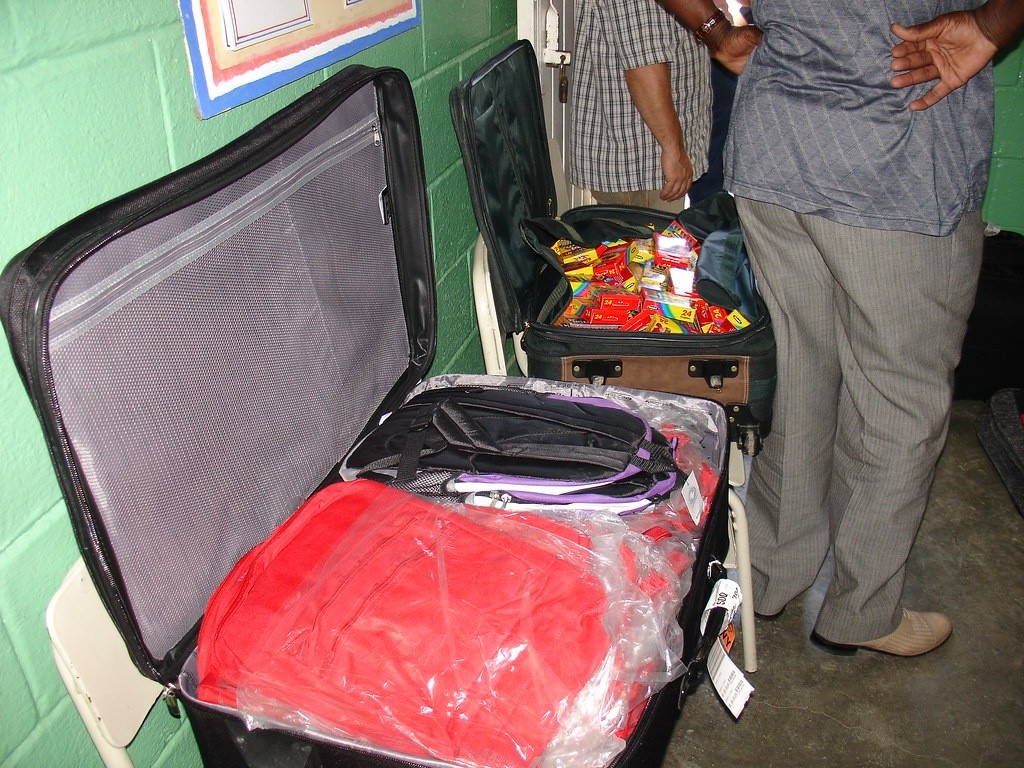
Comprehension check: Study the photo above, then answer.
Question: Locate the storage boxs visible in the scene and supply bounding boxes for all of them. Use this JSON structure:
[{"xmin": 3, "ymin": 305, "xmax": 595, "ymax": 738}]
[{"xmin": 550, "ymin": 217, "xmax": 752, "ymax": 336}]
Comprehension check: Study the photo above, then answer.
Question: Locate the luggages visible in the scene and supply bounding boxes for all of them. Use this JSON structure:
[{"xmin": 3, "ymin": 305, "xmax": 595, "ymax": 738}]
[
  {"xmin": 0, "ymin": 64, "xmax": 731, "ymax": 768},
  {"xmin": 450, "ymin": 40, "xmax": 777, "ymax": 422}
]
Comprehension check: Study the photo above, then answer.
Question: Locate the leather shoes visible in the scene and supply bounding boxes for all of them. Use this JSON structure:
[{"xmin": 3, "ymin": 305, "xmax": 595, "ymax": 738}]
[
  {"xmin": 753, "ymin": 605, "xmax": 786, "ymax": 621},
  {"xmin": 810, "ymin": 607, "xmax": 953, "ymax": 657}
]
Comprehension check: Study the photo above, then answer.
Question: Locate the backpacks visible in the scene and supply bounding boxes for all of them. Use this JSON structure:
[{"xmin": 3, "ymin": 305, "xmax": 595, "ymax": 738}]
[{"xmin": 196, "ymin": 385, "xmax": 717, "ymax": 766}]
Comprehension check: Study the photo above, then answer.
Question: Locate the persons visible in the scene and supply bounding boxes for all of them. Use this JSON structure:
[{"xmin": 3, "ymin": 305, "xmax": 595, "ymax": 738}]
[
  {"xmin": 660, "ymin": 0, "xmax": 1023, "ymax": 659},
  {"xmin": 576, "ymin": 2, "xmax": 713, "ymax": 215}
]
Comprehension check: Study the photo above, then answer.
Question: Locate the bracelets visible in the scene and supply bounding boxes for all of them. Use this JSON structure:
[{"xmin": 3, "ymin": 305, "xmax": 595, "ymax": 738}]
[{"xmin": 692, "ymin": 10, "xmax": 725, "ymax": 41}]
